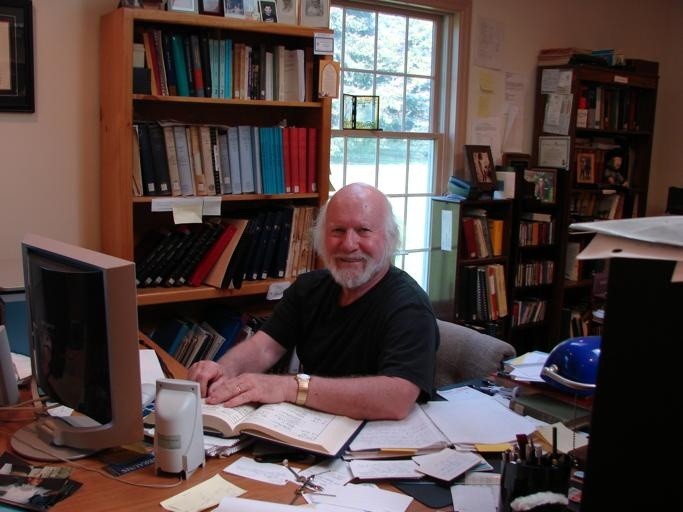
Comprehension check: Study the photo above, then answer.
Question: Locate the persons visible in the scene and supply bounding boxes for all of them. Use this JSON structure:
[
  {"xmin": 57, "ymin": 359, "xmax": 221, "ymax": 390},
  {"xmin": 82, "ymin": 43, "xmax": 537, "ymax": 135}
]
[
  {"xmin": 0, "ymin": 477, "xmax": 59, "ymax": 504},
  {"xmin": 605, "ymin": 150, "xmax": 628, "ymax": 188},
  {"xmin": 281, "ymin": 0, "xmax": 291, "ymax": 12},
  {"xmin": 579, "ymin": 156, "xmax": 591, "ymax": 180},
  {"xmin": 477, "ymin": 152, "xmax": 487, "ymax": 182},
  {"xmin": 261, "ymin": 4, "xmax": 276, "ymax": 22},
  {"xmin": 308, "ymin": 0, "xmax": 322, "ymax": 15},
  {"xmin": 532, "ymin": 176, "xmax": 551, "ymax": 202}
]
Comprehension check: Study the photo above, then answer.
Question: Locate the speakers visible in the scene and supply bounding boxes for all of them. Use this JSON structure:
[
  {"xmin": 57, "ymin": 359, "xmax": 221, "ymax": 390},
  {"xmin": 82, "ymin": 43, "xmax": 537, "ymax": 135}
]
[
  {"xmin": 155, "ymin": 379, "xmax": 205, "ymax": 479},
  {"xmin": 0, "ymin": 326, "xmax": 19, "ymax": 405}
]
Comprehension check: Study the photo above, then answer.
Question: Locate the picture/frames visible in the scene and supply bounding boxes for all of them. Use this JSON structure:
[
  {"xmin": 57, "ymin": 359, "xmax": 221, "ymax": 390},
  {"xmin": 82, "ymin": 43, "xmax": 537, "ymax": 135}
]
[
  {"xmin": 1, "ymin": 0, "xmax": 37, "ymax": 115},
  {"xmin": 342, "ymin": 91, "xmax": 382, "ymax": 133},
  {"xmin": 464, "ymin": 143, "xmax": 498, "ymax": 192},
  {"xmin": 527, "ymin": 167, "xmax": 558, "ymax": 205},
  {"xmin": 574, "ymin": 145, "xmax": 598, "ymax": 186},
  {"xmin": 117, "ymin": 0, "xmax": 331, "ymax": 27}
]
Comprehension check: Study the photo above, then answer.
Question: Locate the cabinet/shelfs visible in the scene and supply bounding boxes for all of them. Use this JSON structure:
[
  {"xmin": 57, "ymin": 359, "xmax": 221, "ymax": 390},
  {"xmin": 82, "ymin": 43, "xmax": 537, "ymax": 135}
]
[
  {"xmin": 431, "ymin": 56, "xmax": 662, "ymax": 353},
  {"xmin": 97, "ymin": 6, "xmax": 336, "ymax": 393}
]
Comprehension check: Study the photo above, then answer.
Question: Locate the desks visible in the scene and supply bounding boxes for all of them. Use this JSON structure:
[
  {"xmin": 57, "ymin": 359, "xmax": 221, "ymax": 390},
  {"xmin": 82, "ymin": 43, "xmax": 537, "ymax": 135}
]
[{"xmin": 0, "ymin": 331, "xmax": 629, "ymax": 512}]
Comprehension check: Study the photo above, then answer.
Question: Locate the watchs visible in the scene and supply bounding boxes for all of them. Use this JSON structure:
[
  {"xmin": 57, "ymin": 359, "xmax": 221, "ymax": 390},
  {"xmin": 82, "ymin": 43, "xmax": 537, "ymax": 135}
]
[{"xmin": 294, "ymin": 372, "xmax": 311, "ymax": 405}]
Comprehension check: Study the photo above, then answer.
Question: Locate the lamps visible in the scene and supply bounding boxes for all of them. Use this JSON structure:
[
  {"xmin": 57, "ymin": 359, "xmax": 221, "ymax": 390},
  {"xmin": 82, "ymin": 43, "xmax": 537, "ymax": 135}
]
[{"xmin": 541, "ymin": 333, "xmax": 602, "ymax": 467}]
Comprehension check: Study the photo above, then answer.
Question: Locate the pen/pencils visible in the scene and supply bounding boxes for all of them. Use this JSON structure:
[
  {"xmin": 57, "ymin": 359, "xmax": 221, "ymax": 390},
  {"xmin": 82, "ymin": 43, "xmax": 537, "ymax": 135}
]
[{"xmin": 501, "ymin": 427, "xmax": 557, "ymax": 466}]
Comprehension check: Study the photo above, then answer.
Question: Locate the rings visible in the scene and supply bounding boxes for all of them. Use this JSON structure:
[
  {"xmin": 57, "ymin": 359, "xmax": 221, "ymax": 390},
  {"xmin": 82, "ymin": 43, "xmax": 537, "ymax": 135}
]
[{"xmin": 236, "ymin": 384, "xmax": 243, "ymax": 393}]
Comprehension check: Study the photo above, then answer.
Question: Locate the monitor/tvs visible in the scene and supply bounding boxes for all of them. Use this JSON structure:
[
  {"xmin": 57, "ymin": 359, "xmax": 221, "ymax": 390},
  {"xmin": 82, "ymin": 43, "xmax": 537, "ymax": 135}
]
[{"xmin": 10, "ymin": 233, "xmax": 144, "ymax": 462}]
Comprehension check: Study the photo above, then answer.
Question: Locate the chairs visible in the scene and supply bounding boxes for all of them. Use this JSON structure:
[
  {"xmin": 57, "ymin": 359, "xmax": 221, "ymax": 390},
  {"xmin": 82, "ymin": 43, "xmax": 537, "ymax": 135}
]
[{"xmin": 422, "ymin": 307, "xmax": 513, "ymax": 396}]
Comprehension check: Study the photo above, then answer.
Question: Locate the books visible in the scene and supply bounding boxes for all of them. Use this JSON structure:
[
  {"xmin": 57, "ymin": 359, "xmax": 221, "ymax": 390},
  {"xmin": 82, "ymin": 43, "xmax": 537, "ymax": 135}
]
[
  {"xmin": 343, "ymin": 387, "xmax": 539, "ymax": 462},
  {"xmin": 512, "ymin": 299, "xmax": 546, "ymax": 326},
  {"xmin": 571, "ymin": 194, "xmax": 625, "ymax": 227},
  {"xmin": 568, "ymin": 241, "xmax": 587, "ymax": 283},
  {"xmin": 200, "ymin": 393, "xmax": 369, "ymax": 460},
  {"xmin": 537, "ymin": 46, "xmax": 607, "ymax": 67},
  {"xmin": 520, "ymin": 213, "xmax": 553, "ymax": 246},
  {"xmin": 142, "ymin": 30, "xmax": 314, "ymax": 103},
  {"xmin": 464, "ymin": 215, "xmax": 505, "ymax": 263},
  {"xmin": 146, "ymin": 313, "xmax": 273, "ymax": 367},
  {"xmin": 584, "ymin": 85, "xmax": 637, "ymax": 131},
  {"xmin": 497, "ymin": 350, "xmax": 551, "ymax": 380},
  {"xmin": 137, "ymin": 206, "xmax": 317, "ymax": 290},
  {"xmin": 467, "ymin": 264, "xmax": 508, "ymax": 323},
  {"xmin": 564, "ymin": 308, "xmax": 604, "ymax": 336},
  {"xmin": 132, "ymin": 122, "xmax": 317, "ymax": 197},
  {"xmin": 514, "ymin": 262, "xmax": 554, "ymax": 287}
]
